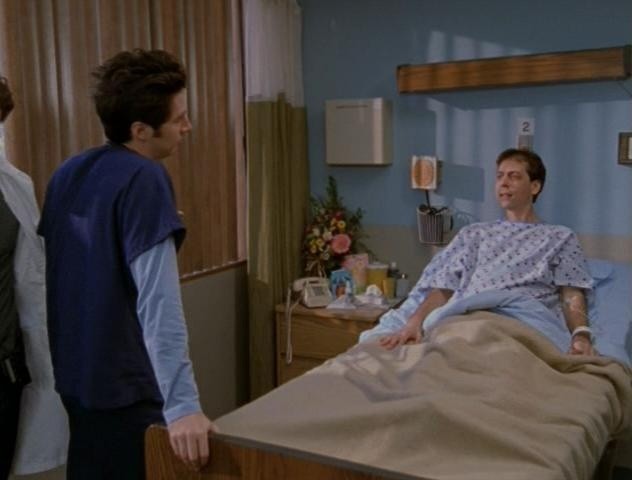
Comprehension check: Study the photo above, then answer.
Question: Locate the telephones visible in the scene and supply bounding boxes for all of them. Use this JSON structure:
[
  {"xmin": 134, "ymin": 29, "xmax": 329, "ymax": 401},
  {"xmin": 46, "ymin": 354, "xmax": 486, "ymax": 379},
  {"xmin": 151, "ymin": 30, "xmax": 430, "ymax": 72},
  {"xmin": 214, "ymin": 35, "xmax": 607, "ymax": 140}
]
[{"xmin": 293, "ymin": 277, "xmax": 333, "ymax": 308}]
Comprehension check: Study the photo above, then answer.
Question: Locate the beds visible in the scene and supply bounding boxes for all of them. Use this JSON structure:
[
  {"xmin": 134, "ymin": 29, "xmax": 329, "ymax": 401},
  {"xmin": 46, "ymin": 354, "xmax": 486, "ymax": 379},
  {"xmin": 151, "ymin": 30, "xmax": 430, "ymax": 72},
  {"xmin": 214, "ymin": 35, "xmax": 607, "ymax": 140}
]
[{"xmin": 143, "ymin": 262, "xmax": 632, "ymax": 479}]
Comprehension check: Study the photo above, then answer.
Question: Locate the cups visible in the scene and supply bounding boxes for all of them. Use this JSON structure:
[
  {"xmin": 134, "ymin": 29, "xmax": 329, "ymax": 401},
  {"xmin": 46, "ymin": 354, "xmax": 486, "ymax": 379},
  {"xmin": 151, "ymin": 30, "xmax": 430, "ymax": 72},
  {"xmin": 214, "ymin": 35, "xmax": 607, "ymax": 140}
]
[
  {"xmin": 383, "ymin": 278, "xmax": 397, "ymax": 298},
  {"xmin": 365, "ymin": 262, "xmax": 388, "ymax": 297}
]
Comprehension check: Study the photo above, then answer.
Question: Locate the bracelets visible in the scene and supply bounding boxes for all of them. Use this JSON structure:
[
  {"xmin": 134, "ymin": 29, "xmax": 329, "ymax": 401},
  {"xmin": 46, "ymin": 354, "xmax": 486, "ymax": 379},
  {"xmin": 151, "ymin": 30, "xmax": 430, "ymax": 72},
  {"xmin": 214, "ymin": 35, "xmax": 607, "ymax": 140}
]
[{"xmin": 568, "ymin": 324, "xmax": 598, "ymax": 344}]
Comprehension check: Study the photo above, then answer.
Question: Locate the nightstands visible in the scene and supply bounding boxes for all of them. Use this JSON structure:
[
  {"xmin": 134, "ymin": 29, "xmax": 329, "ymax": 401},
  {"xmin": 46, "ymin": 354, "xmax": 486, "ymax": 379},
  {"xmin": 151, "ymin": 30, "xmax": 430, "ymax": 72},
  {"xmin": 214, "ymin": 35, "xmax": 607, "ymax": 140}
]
[{"xmin": 274, "ymin": 296, "xmax": 407, "ymax": 389}]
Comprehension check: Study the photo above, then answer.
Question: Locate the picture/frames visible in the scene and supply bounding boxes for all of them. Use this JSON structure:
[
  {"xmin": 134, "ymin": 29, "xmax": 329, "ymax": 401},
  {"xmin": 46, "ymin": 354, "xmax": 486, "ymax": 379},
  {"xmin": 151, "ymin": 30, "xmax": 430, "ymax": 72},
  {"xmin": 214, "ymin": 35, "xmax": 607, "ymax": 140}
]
[{"xmin": 618, "ymin": 131, "xmax": 632, "ymax": 164}]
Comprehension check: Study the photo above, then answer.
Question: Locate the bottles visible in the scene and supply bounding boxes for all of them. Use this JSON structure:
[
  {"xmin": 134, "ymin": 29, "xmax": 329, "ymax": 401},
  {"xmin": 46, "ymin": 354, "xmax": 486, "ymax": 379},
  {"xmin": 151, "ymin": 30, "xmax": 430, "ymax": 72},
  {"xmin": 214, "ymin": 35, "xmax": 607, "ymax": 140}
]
[
  {"xmin": 388, "ymin": 262, "xmax": 401, "ymax": 277},
  {"xmin": 396, "ymin": 273, "xmax": 408, "ymax": 297}
]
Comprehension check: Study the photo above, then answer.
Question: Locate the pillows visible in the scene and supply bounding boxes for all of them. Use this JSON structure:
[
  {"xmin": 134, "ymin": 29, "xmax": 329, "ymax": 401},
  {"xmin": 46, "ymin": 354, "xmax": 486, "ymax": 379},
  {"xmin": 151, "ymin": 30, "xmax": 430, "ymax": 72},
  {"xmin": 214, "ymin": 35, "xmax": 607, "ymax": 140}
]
[{"xmin": 583, "ymin": 258, "xmax": 615, "ymax": 289}]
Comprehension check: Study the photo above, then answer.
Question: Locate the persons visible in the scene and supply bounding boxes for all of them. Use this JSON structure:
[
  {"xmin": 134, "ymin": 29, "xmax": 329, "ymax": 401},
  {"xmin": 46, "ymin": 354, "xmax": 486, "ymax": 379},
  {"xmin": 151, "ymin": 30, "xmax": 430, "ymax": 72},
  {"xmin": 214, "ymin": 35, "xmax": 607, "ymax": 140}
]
[
  {"xmin": 377, "ymin": 144, "xmax": 602, "ymax": 373},
  {"xmin": 0, "ymin": 70, "xmax": 63, "ymax": 478},
  {"xmin": 36, "ymin": 47, "xmax": 217, "ymax": 478}
]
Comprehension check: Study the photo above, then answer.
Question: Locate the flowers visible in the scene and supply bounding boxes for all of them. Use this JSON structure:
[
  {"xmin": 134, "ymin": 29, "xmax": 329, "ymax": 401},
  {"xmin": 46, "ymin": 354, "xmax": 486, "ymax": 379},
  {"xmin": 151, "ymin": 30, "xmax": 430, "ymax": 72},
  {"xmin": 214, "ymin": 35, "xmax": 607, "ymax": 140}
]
[{"xmin": 304, "ymin": 176, "xmax": 378, "ymax": 277}]
